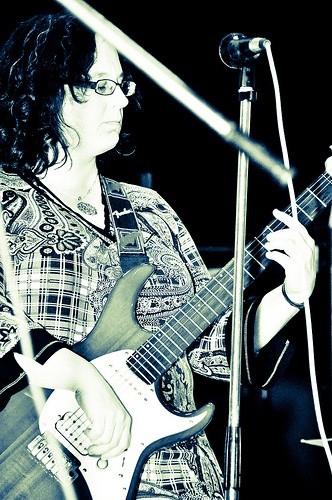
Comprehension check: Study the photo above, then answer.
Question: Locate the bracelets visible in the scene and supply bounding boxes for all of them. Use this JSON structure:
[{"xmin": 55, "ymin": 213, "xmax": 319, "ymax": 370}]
[{"xmin": 279, "ymin": 281, "xmax": 305, "ymax": 311}]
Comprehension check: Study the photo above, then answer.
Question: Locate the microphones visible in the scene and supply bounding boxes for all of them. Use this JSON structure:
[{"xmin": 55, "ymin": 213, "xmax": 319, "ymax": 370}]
[{"xmin": 218, "ymin": 33, "xmax": 271, "ymax": 69}]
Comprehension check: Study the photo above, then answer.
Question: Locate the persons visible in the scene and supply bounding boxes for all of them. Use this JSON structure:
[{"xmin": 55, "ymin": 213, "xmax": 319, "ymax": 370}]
[{"xmin": 0, "ymin": 11, "xmax": 318, "ymax": 500}]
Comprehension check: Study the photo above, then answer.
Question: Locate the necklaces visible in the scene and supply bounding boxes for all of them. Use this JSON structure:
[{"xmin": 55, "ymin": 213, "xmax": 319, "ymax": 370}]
[{"xmin": 75, "ymin": 186, "xmax": 99, "ymax": 216}]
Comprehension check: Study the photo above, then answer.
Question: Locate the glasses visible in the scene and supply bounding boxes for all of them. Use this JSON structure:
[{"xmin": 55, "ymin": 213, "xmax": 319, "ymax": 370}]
[{"xmin": 62, "ymin": 78, "xmax": 137, "ymax": 97}]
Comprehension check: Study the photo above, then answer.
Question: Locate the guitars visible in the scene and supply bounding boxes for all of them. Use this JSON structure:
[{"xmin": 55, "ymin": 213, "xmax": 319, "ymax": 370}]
[{"xmin": 1, "ymin": 142, "xmax": 332, "ymax": 499}]
